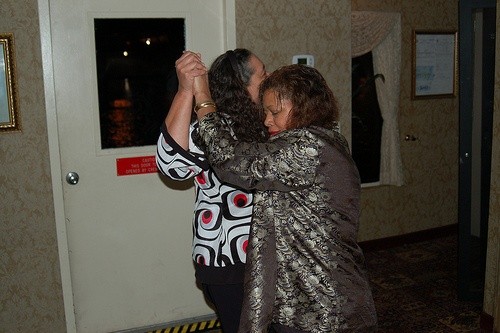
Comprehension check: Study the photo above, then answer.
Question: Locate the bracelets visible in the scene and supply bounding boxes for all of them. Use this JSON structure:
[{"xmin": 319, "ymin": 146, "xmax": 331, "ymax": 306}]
[{"xmin": 193, "ymin": 102, "xmax": 216, "ymax": 112}]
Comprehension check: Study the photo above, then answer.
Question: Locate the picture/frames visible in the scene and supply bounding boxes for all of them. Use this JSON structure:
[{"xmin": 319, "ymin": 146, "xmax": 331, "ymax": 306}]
[
  {"xmin": 411, "ymin": 28, "xmax": 459, "ymax": 101},
  {"xmin": 0, "ymin": 33, "xmax": 23, "ymax": 133}
]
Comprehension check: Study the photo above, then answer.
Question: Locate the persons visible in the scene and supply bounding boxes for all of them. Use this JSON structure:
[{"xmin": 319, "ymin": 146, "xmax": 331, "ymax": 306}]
[
  {"xmin": 154, "ymin": 49, "xmax": 287, "ymax": 333},
  {"xmin": 184, "ymin": 51, "xmax": 380, "ymax": 333}
]
